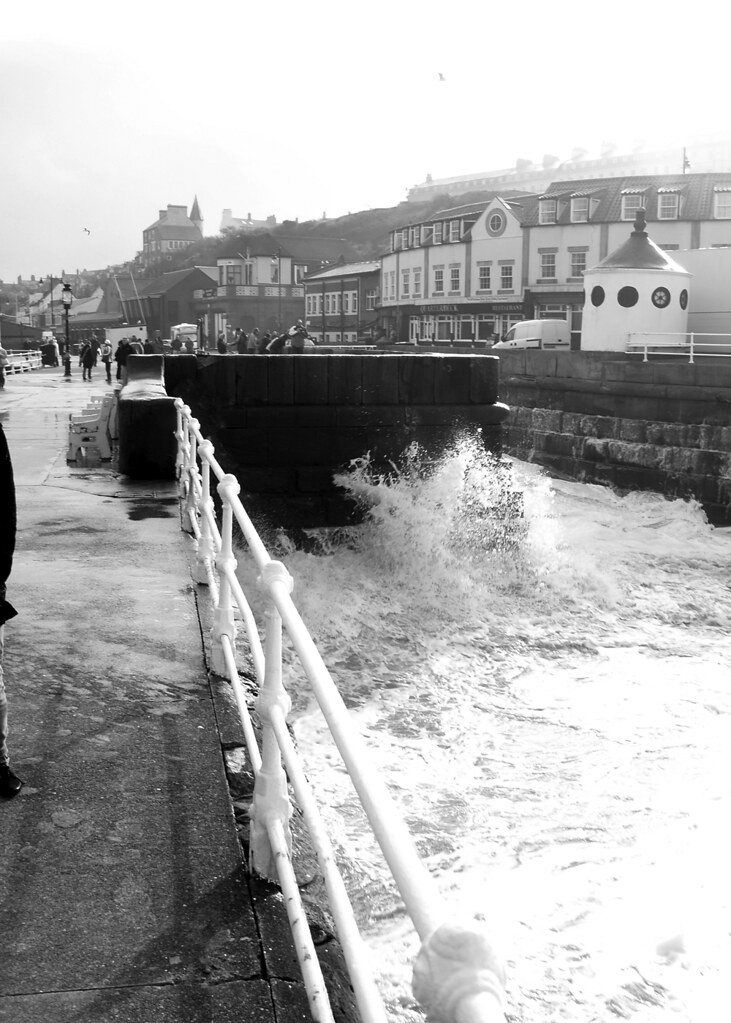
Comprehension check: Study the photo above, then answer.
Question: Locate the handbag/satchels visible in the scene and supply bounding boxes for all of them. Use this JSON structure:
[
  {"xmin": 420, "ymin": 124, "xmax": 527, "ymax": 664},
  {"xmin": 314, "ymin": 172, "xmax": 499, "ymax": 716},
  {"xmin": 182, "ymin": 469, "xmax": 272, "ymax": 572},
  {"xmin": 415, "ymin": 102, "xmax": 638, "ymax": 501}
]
[{"xmin": 101, "ymin": 356, "xmax": 109, "ymax": 363}]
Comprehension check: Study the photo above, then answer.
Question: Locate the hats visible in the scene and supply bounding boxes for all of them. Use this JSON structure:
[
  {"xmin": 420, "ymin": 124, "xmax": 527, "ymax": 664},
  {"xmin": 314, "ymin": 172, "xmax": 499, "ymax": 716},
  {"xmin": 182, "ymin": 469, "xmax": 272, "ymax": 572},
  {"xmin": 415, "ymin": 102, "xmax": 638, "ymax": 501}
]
[
  {"xmin": 120, "ymin": 336, "xmax": 129, "ymax": 341},
  {"xmin": 105, "ymin": 340, "xmax": 111, "ymax": 344}
]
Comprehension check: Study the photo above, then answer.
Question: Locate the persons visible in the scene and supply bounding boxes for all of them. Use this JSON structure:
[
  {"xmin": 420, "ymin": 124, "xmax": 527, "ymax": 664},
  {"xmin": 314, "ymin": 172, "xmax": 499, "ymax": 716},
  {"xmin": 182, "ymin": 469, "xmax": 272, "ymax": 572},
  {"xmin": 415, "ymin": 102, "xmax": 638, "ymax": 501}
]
[
  {"xmin": 0, "ymin": 426, "xmax": 25, "ymax": 800},
  {"xmin": 0, "ymin": 346, "xmax": 9, "ymax": 391},
  {"xmin": 481, "ymin": 330, "xmax": 502, "ymax": 353},
  {"xmin": 22, "ymin": 307, "xmax": 323, "ymax": 383},
  {"xmin": 370, "ymin": 322, "xmax": 399, "ymax": 347}
]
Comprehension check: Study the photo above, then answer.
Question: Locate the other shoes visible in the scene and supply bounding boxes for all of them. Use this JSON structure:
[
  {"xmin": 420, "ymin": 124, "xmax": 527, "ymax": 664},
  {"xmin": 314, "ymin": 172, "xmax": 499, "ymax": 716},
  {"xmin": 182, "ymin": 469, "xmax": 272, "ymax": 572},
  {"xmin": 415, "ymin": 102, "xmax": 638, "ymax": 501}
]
[
  {"xmin": 0, "ymin": 387, "xmax": 4, "ymax": 391},
  {"xmin": 105, "ymin": 378, "xmax": 111, "ymax": 381},
  {"xmin": 89, "ymin": 376, "xmax": 91, "ymax": 379},
  {"xmin": 83, "ymin": 378, "xmax": 86, "ymax": 381},
  {"xmin": 0, "ymin": 763, "xmax": 25, "ymax": 800}
]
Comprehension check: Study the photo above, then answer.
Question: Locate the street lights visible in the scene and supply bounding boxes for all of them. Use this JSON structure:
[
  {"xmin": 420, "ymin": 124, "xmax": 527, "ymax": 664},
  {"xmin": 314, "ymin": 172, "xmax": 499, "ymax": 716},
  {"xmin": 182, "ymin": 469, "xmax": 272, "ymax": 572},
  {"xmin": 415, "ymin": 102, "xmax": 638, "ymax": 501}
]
[
  {"xmin": 271, "ymin": 247, "xmax": 283, "ymax": 337},
  {"xmin": 61, "ymin": 282, "xmax": 73, "ymax": 377}
]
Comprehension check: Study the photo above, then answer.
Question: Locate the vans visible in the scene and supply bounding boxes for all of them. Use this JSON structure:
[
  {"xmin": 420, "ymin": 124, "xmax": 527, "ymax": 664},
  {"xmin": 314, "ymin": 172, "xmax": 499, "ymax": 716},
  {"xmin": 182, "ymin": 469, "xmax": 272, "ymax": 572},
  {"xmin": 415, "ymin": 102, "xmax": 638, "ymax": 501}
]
[{"xmin": 491, "ymin": 319, "xmax": 572, "ymax": 350}]
